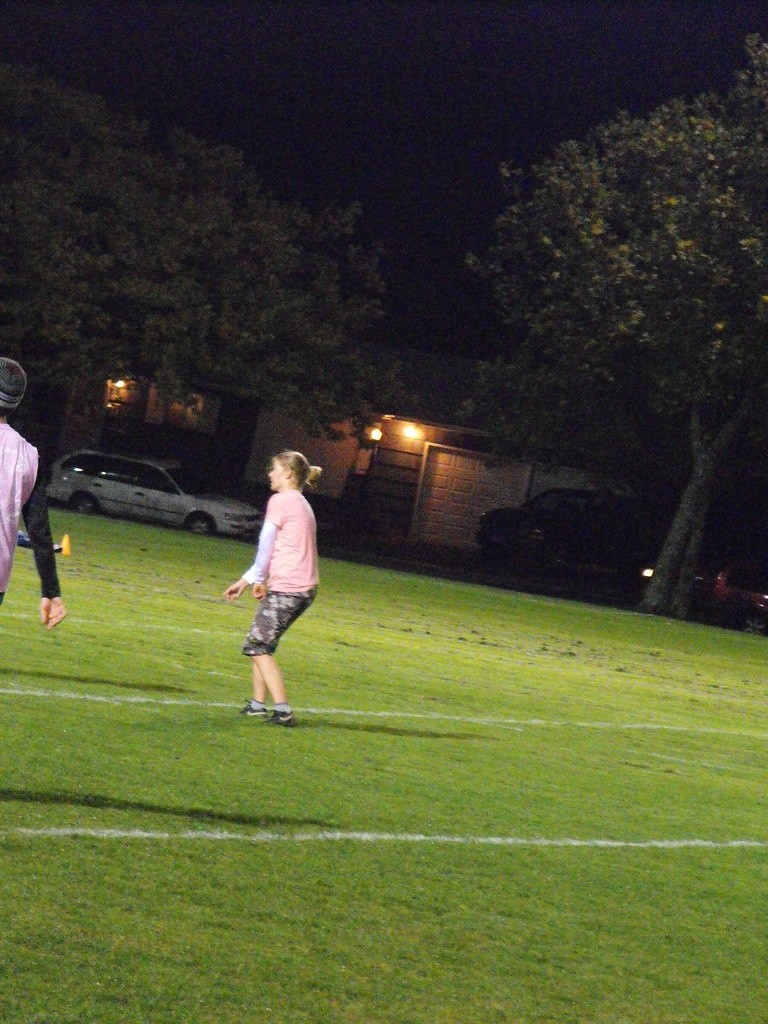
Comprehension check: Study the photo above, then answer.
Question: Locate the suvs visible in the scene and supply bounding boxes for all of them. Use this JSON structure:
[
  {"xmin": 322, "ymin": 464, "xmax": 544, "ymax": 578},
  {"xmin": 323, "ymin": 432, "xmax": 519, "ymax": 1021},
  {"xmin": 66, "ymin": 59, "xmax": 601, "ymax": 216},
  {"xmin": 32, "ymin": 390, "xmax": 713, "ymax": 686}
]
[{"xmin": 475, "ymin": 490, "xmax": 668, "ymax": 594}]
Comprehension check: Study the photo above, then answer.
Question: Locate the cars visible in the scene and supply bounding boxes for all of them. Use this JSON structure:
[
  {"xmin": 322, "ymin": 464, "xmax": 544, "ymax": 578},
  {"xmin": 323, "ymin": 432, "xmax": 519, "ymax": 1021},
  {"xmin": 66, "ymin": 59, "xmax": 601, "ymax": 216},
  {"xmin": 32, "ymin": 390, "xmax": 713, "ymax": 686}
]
[
  {"xmin": 640, "ymin": 555, "xmax": 767, "ymax": 635},
  {"xmin": 44, "ymin": 447, "xmax": 263, "ymax": 537}
]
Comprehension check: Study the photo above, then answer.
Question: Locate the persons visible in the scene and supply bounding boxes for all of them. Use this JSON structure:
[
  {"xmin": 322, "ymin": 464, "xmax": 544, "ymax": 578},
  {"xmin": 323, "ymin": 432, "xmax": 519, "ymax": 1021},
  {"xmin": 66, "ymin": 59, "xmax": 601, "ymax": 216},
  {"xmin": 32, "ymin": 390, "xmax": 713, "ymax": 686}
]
[
  {"xmin": 219, "ymin": 451, "xmax": 323, "ymax": 727},
  {"xmin": 1, "ymin": 357, "xmax": 66, "ymax": 629}
]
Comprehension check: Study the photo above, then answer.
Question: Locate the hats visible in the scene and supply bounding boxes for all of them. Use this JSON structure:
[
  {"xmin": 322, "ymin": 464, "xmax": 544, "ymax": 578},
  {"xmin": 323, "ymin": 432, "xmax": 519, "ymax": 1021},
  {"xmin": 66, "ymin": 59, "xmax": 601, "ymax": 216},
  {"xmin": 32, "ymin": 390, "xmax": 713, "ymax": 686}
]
[{"xmin": 1, "ymin": 357, "xmax": 27, "ymax": 409}]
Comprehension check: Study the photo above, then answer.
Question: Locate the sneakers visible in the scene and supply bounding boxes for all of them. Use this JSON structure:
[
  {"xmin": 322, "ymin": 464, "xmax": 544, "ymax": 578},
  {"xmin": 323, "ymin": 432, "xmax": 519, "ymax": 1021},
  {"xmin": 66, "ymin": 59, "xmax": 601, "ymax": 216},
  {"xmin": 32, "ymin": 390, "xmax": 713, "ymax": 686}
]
[
  {"xmin": 240, "ymin": 704, "xmax": 267, "ymax": 717},
  {"xmin": 262, "ymin": 712, "xmax": 299, "ymax": 728}
]
